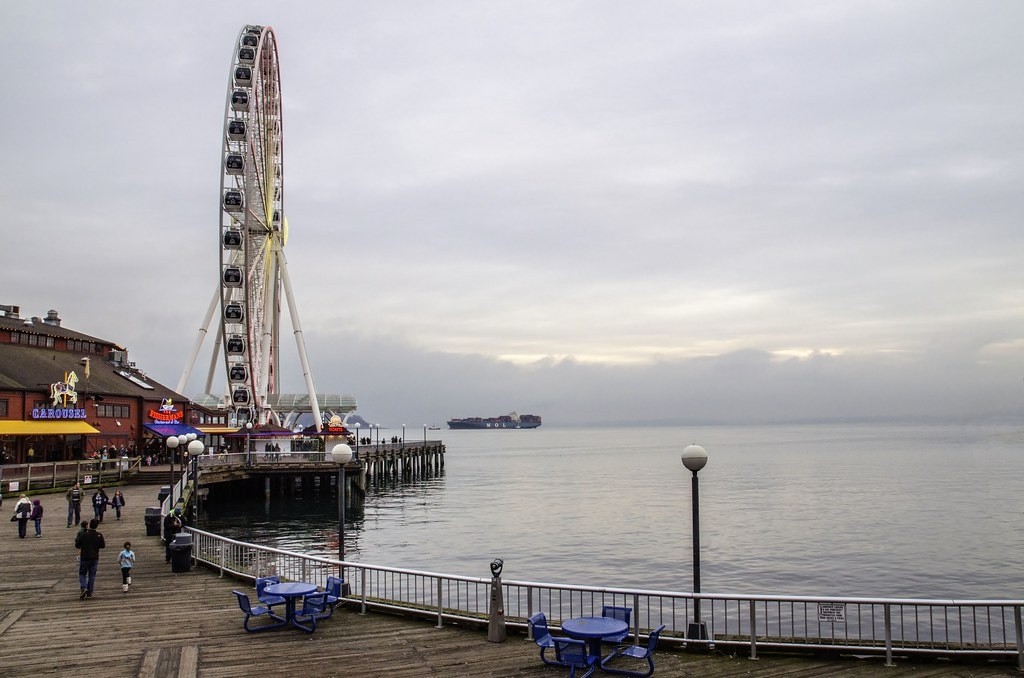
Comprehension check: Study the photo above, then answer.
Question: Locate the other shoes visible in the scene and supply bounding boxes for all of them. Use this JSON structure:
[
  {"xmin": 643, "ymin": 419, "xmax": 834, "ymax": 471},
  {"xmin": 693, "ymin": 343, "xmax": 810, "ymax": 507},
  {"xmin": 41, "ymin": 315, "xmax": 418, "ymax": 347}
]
[
  {"xmin": 87, "ymin": 594, "xmax": 92, "ymax": 599},
  {"xmin": 99, "ymin": 520, "xmax": 104, "ymax": 524},
  {"xmin": 75, "ymin": 522, "xmax": 80, "ymax": 526},
  {"xmin": 67, "ymin": 525, "xmax": 72, "ymax": 528},
  {"xmin": 116, "ymin": 518, "xmax": 120, "ymax": 521},
  {"xmin": 35, "ymin": 533, "xmax": 42, "ymax": 538},
  {"xmin": 20, "ymin": 535, "xmax": 28, "ymax": 538},
  {"xmin": 80, "ymin": 589, "xmax": 86, "ymax": 599}
]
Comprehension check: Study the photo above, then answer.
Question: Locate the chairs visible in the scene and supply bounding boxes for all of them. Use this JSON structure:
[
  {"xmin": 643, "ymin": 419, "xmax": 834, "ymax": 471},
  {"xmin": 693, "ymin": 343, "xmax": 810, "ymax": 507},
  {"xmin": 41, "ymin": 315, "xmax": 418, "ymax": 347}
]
[
  {"xmin": 232, "ymin": 576, "xmax": 343, "ymax": 633},
  {"xmin": 527, "ymin": 606, "xmax": 666, "ymax": 678}
]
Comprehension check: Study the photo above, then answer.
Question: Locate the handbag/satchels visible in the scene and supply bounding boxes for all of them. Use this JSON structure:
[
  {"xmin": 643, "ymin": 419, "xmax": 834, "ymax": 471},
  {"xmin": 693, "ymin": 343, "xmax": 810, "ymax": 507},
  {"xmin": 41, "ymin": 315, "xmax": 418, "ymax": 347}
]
[{"xmin": 11, "ymin": 515, "xmax": 18, "ymax": 521}]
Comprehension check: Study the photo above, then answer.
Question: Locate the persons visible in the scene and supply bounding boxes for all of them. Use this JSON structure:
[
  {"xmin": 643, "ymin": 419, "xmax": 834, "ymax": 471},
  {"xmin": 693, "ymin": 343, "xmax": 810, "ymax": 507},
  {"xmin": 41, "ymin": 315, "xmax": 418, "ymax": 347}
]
[
  {"xmin": 291, "ymin": 437, "xmax": 325, "ymax": 460},
  {"xmin": 216, "ymin": 445, "xmax": 231, "ymax": 463},
  {"xmin": 92, "ymin": 488, "xmax": 114, "ymax": 522},
  {"xmin": 112, "ymin": 491, "xmax": 125, "ymax": 520},
  {"xmin": 28, "ymin": 446, "xmax": 34, "ymax": 464},
  {"xmin": 75, "ymin": 519, "xmax": 105, "ymax": 598},
  {"xmin": 382, "ymin": 438, "xmax": 385, "ymax": 444},
  {"xmin": 93, "ymin": 443, "xmax": 198, "ymax": 467},
  {"xmin": 361, "ymin": 437, "xmax": 371, "ymax": 445},
  {"xmin": 347, "ymin": 436, "xmax": 356, "ymax": 445},
  {"xmin": 391, "ymin": 436, "xmax": 397, "ymax": 443},
  {"xmin": 65, "ymin": 482, "xmax": 85, "ymax": 528},
  {"xmin": 118, "ymin": 541, "xmax": 135, "ymax": 591},
  {"xmin": 30, "ymin": 499, "xmax": 43, "ymax": 537},
  {"xmin": 14, "ymin": 493, "xmax": 32, "ymax": 540},
  {"xmin": 399, "ymin": 438, "xmax": 402, "ymax": 443},
  {"xmin": 264, "ymin": 443, "xmax": 281, "ymax": 463}
]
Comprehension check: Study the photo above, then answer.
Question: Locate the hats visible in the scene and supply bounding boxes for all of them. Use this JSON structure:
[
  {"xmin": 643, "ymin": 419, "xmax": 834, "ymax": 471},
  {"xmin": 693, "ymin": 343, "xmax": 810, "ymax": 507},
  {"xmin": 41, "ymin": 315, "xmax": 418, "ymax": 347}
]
[
  {"xmin": 175, "ymin": 507, "xmax": 182, "ymax": 513},
  {"xmin": 102, "ymin": 444, "xmax": 108, "ymax": 448},
  {"xmin": 110, "ymin": 445, "xmax": 115, "ymax": 448}
]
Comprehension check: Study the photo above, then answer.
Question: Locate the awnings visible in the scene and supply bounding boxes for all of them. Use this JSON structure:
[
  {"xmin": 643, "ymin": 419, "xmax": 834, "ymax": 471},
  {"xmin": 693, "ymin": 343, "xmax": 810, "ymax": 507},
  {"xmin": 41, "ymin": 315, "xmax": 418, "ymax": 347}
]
[
  {"xmin": 143, "ymin": 424, "xmax": 205, "ymax": 439},
  {"xmin": 0, "ymin": 420, "xmax": 101, "ymax": 435}
]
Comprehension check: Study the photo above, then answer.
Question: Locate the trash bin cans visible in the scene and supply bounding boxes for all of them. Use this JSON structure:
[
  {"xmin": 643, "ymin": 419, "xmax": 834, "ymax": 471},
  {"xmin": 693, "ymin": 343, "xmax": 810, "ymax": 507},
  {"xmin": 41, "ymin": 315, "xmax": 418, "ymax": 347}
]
[
  {"xmin": 158, "ymin": 492, "xmax": 169, "ymax": 507},
  {"xmin": 169, "ymin": 542, "xmax": 194, "ymax": 573},
  {"xmin": 144, "ymin": 514, "xmax": 161, "ymax": 536},
  {"xmin": 161, "ymin": 487, "xmax": 170, "ymax": 493}
]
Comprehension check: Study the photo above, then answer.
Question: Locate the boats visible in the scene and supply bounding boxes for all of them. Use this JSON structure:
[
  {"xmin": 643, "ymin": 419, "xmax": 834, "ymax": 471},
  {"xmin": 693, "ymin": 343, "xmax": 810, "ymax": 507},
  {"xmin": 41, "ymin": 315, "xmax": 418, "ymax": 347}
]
[
  {"xmin": 447, "ymin": 411, "xmax": 542, "ymax": 429},
  {"xmin": 428, "ymin": 426, "xmax": 441, "ymax": 430}
]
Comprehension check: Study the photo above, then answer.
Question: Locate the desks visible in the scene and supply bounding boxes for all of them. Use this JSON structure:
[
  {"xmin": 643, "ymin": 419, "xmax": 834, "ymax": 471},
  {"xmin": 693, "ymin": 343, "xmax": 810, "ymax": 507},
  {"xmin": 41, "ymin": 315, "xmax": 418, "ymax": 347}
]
[
  {"xmin": 562, "ymin": 617, "xmax": 629, "ymax": 664},
  {"xmin": 263, "ymin": 583, "xmax": 318, "ymax": 626}
]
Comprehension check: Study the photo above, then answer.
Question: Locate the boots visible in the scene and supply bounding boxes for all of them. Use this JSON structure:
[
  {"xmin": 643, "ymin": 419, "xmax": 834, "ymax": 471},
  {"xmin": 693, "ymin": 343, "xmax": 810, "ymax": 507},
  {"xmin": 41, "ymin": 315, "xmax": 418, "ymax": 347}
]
[
  {"xmin": 126, "ymin": 576, "xmax": 132, "ymax": 585},
  {"xmin": 121, "ymin": 584, "xmax": 129, "ymax": 592}
]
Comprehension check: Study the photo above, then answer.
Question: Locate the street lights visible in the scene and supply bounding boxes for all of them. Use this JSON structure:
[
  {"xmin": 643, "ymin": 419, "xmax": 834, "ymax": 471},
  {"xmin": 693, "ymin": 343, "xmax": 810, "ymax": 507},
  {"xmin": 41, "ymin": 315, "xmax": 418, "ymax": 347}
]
[
  {"xmin": 187, "ymin": 440, "xmax": 205, "ymax": 529},
  {"xmin": 166, "ymin": 435, "xmax": 180, "ymax": 512},
  {"xmin": 246, "ymin": 423, "xmax": 252, "ymax": 465},
  {"xmin": 178, "ymin": 435, "xmax": 188, "ymax": 502},
  {"xmin": 424, "ymin": 424, "xmax": 426, "ymax": 446},
  {"xmin": 681, "ymin": 445, "xmax": 709, "ymax": 652},
  {"xmin": 354, "ymin": 422, "xmax": 361, "ymax": 464},
  {"xmin": 402, "ymin": 424, "xmax": 406, "ymax": 448},
  {"xmin": 331, "ymin": 443, "xmax": 352, "ymax": 597},
  {"xmin": 376, "ymin": 423, "xmax": 379, "ymax": 451},
  {"xmin": 186, "ymin": 432, "xmax": 197, "ymax": 480},
  {"xmin": 369, "ymin": 424, "xmax": 373, "ymax": 439}
]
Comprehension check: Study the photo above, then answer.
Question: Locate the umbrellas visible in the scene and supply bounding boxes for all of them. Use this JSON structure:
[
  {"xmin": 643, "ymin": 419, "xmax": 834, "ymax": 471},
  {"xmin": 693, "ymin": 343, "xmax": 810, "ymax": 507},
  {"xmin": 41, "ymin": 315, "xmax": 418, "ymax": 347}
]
[
  {"xmin": 302, "ymin": 422, "xmax": 353, "ymax": 452},
  {"xmin": 226, "ymin": 423, "xmax": 296, "ymax": 452}
]
